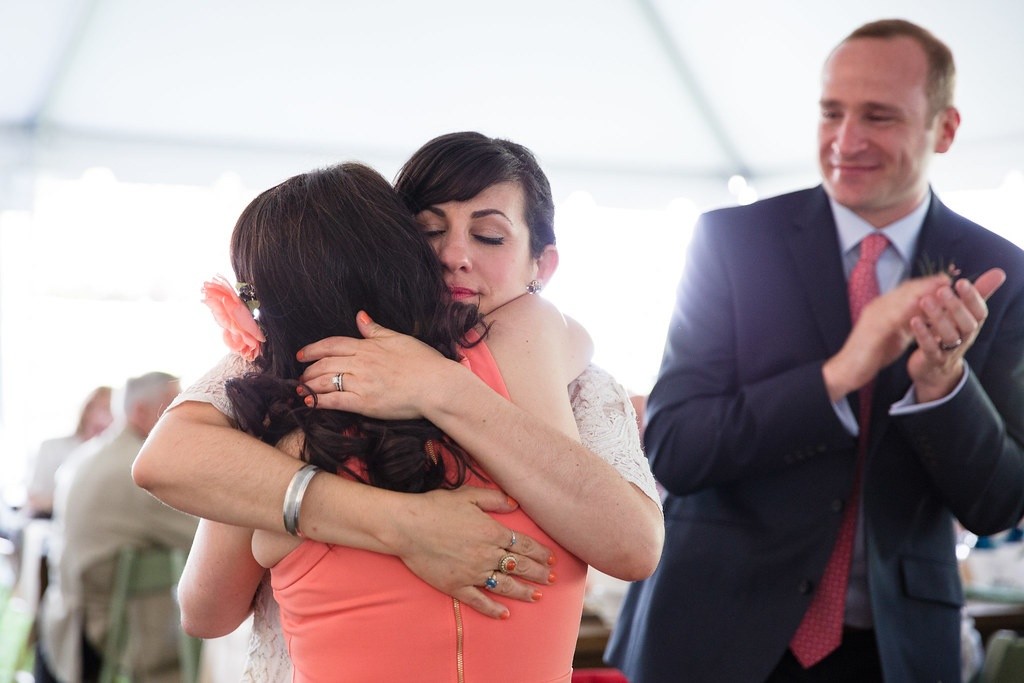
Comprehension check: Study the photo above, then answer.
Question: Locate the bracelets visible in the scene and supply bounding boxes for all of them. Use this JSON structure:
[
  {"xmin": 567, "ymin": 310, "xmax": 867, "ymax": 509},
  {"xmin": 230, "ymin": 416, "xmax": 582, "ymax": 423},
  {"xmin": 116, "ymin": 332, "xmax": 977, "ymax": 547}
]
[{"xmin": 283, "ymin": 463, "xmax": 322, "ymax": 538}]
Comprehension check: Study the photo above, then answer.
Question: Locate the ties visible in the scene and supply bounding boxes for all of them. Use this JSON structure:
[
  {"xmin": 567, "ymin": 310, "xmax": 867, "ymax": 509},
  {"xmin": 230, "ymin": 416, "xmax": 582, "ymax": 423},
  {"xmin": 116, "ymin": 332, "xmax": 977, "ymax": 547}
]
[{"xmin": 786, "ymin": 233, "xmax": 890, "ymax": 666}]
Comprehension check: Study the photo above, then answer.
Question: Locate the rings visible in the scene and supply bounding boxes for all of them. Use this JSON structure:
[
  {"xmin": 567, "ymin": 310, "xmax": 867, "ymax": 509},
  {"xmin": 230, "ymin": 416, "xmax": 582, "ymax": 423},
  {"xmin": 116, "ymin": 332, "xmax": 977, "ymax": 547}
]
[
  {"xmin": 485, "ymin": 571, "xmax": 498, "ymax": 590},
  {"xmin": 939, "ymin": 340, "xmax": 962, "ymax": 349},
  {"xmin": 505, "ymin": 530, "xmax": 516, "ymax": 551},
  {"xmin": 498, "ymin": 552, "xmax": 517, "ymax": 575},
  {"xmin": 332, "ymin": 373, "xmax": 344, "ymax": 392}
]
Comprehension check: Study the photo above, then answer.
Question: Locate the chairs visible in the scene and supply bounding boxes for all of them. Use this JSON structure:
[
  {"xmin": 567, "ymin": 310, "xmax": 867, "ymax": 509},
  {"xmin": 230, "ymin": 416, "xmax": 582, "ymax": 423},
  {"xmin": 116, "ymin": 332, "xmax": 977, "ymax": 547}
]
[{"xmin": 96, "ymin": 542, "xmax": 198, "ymax": 683}]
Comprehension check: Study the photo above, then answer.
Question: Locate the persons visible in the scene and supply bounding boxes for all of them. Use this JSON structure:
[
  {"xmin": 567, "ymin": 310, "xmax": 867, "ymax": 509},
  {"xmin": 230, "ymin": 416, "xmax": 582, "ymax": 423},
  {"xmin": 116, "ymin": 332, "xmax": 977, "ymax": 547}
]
[
  {"xmin": 14, "ymin": 131, "xmax": 665, "ymax": 683},
  {"xmin": 603, "ymin": 20, "xmax": 1024, "ymax": 683}
]
